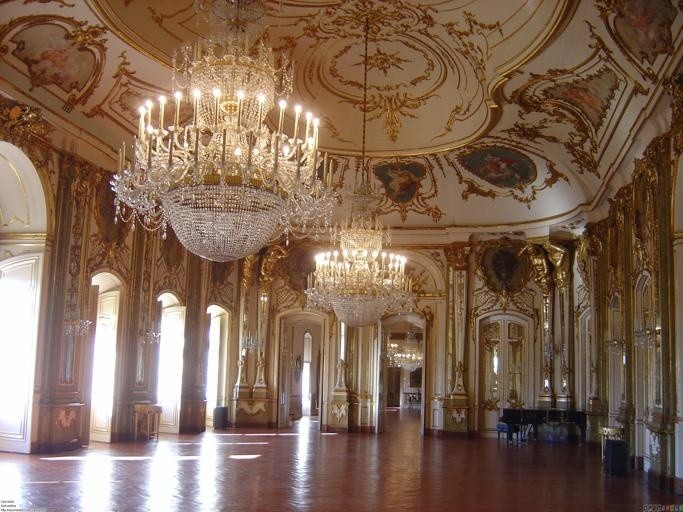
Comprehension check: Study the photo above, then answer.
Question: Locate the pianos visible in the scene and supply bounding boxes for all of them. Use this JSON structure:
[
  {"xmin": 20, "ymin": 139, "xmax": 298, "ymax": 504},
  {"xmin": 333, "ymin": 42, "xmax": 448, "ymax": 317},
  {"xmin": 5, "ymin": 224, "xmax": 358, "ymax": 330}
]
[{"xmin": 498, "ymin": 408, "xmax": 587, "ymax": 445}]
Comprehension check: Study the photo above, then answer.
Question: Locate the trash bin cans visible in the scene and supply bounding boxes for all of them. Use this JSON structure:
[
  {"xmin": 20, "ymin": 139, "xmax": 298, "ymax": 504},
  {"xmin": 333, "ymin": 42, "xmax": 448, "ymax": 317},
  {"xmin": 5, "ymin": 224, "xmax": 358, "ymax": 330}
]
[
  {"xmin": 604, "ymin": 439, "xmax": 627, "ymax": 477},
  {"xmin": 214, "ymin": 406, "xmax": 227, "ymax": 428}
]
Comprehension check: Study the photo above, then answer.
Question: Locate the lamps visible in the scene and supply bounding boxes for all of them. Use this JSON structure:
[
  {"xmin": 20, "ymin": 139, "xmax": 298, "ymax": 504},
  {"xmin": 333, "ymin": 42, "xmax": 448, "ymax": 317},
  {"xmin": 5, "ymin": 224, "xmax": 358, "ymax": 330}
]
[
  {"xmin": 242, "ymin": 330, "xmax": 259, "ymax": 353},
  {"xmin": 109, "ymin": 0, "xmax": 339, "ymax": 262},
  {"xmin": 303, "ymin": 14, "xmax": 416, "ymax": 328},
  {"xmin": 65, "ymin": 320, "xmax": 92, "ymax": 337},
  {"xmin": 387, "ymin": 325, "xmax": 424, "ymax": 373},
  {"xmin": 145, "ymin": 328, "xmax": 162, "ymax": 345}
]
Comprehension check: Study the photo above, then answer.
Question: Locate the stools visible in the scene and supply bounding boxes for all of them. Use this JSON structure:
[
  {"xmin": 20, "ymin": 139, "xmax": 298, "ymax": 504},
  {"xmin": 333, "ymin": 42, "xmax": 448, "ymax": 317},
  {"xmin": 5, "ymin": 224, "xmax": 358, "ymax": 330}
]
[
  {"xmin": 496, "ymin": 424, "xmax": 518, "ymax": 443},
  {"xmin": 133, "ymin": 404, "xmax": 161, "ymax": 443}
]
